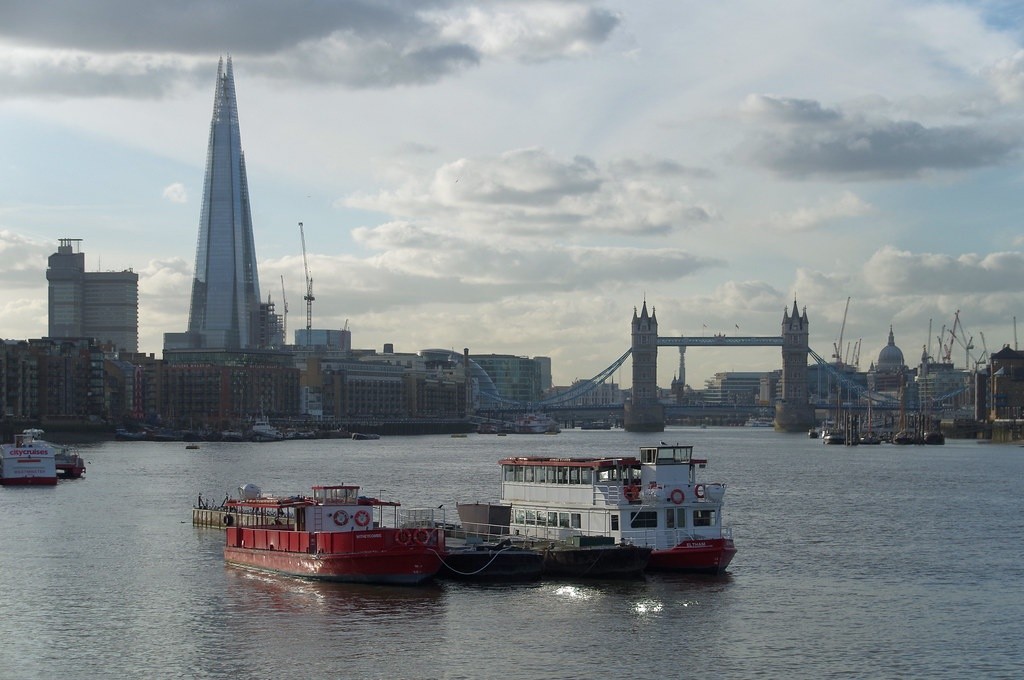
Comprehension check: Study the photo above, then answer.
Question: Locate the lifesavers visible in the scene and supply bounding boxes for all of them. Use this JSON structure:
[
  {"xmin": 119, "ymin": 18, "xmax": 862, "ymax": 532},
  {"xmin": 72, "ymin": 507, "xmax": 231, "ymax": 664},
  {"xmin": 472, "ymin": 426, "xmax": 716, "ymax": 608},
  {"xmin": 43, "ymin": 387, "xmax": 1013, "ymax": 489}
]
[
  {"xmin": 354, "ymin": 510, "xmax": 370, "ymax": 526},
  {"xmin": 223, "ymin": 515, "xmax": 231, "ymax": 526},
  {"xmin": 671, "ymin": 487, "xmax": 685, "ymax": 504},
  {"xmin": 624, "ymin": 484, "xmax": 639, "ymax": 501},
  {"xmin": 394, "ymin": 528, "xmax": 412, "ymax": 545},
  {"xmin": 412, "ymin": 527, "xmax": 430, "ymax": 545},
  {"xmin": 334, "ymin": 510, "xmax": 349, "ymax": 526},
  {"xmin": 694, "ymin": 483, "xmax": 706, "ymax": 498}
]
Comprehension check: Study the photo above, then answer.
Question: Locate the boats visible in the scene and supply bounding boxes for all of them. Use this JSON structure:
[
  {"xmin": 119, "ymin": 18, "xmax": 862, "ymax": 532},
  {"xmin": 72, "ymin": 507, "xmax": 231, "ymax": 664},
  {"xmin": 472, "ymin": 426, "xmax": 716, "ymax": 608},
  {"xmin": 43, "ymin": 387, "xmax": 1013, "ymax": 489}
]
[
  {"xmin": 580, "ymin": 422, "xmax": 612, "ymax": 430},
  {"xmin": 221, "ymin": 490, "xmax": 448, "ymax": 583},
  {"xmin": 498, "ymin": 443, "xmax": 739, "ymax": 575},
  {"xmin": 475, "ymin": 417, "xmax": 562, "ymax": 434},
  {"xmin": 451, "ymin": 433, "xmax": 467, "ymax": 439},
  {"xmin": 894, "ymin": 432, "xmax": 924, "ymax": 444},
  {"xmin": 858, "ymin": 434, "xmax": 882, "ymax": 446},
  {"xmin": 924, "ymin": 430, "xmax": 947, "ymax": 445},
  {"xmin": 498, "ymin": 432, "xmax": 508, "ymax": 437},
  {"xmin": 186, "ymin": 443, "xmax": 200, "ymax": 450},
  {"xmin": 808, "ymin": 427, "xmax": 820, "ymax": 439},
  {"xmin": 823, "ymin": 432, "xmax": 858, "ymax": 445},
  {"xmin": 2, "ymin": 429, "xmax": 94, "ymax": 484},
  {"xmin": 352, "ymin": 431, "xmax": 380, "ymax": 441},
  {"xmin": 543, "ymin": 431, "xmax": 558, "ymax": 435}
]
